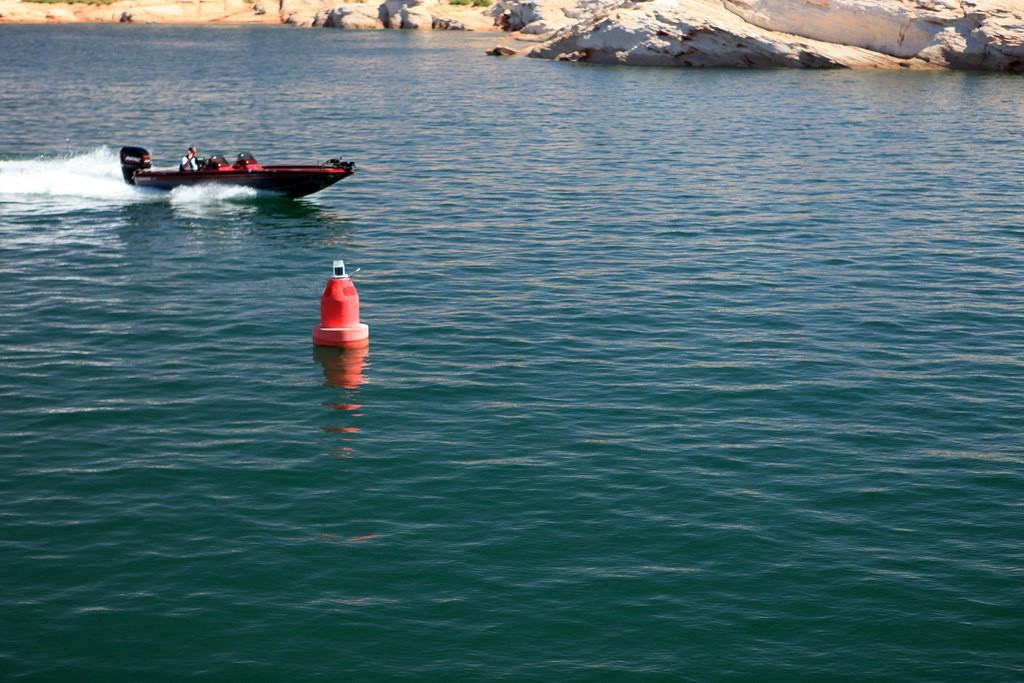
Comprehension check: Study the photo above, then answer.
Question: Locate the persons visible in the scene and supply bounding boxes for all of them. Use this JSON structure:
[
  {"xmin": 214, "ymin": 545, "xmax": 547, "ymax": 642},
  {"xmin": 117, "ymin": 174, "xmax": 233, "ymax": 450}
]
[{"xmin": 180, "ymin": 147, "xmax": 201, "ymax": 171}]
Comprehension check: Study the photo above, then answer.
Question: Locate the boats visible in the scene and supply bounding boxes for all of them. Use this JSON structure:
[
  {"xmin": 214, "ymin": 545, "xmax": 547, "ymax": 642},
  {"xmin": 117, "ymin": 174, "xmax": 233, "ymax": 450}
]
[{"xmin": 118, "ymin": 144, "xmax": 358, "ymax": 199}]
